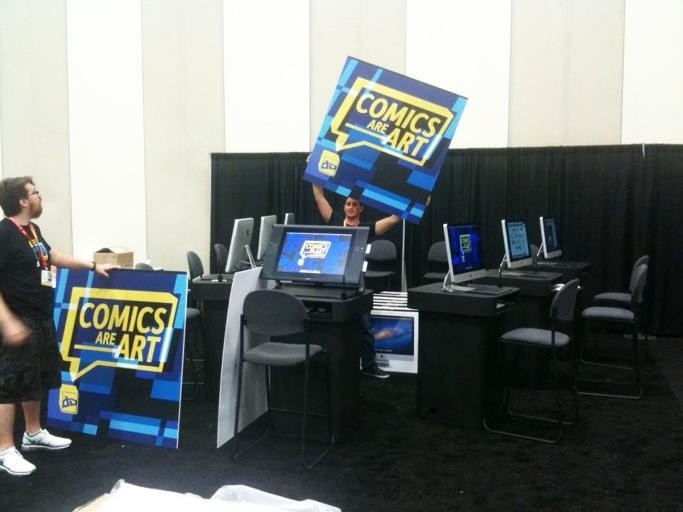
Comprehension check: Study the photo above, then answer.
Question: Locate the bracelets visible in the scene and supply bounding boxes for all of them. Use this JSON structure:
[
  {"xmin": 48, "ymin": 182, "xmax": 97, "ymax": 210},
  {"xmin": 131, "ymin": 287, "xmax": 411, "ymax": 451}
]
[{"xmin": 90, "ymin": 260, "xmax": 96, "ymax": 271}]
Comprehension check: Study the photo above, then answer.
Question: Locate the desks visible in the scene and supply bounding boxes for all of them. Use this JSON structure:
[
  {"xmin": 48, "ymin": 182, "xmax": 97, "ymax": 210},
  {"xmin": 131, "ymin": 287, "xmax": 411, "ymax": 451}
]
[
  {"xmin": 191, "ymin": 260, "xmax": 373, "ymax": 418},
  {"xmin": 407, "ymin": 260, "xmax": 592, "ymax": 422}
]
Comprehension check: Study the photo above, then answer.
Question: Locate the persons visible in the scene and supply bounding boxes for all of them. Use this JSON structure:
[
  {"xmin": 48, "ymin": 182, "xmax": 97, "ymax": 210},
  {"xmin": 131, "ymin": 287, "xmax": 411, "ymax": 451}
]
[
  {"xmin": 0, "ymin": 175, "xmax": 122, "ymax": 476},
  {"xmin": 306, "ymin": 153, "xmax": 431, "ymax": 379}
]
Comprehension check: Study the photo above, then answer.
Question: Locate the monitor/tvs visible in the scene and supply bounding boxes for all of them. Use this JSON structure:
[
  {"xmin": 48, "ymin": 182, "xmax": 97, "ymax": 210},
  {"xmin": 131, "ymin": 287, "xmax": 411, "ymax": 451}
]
[
  {"xmin": 283, "ymin": 211, "xmax": 297, "ymax": 225},
  {"xmin": 254, "ymin": 215, "xmax": 277, "ymax": 259},
  {"xmin": 368, "ymin": 314, "xmax": 415, "ymax": 368},
  {"xmin": 259, "ymin": 222, "xmax": 369, "ymax": 287},
  {"xmin": 223, "ymin": 215, "xmax": 258, "ymax": 271},
  {"xmin": 441, "ymin": 223, "xmax": 487, "ymax": 292},
  {"xmin": 536, "ymin": 215, "xmax": 564, "ymax": 265},
  {"xmin": 496, "ymin": 218, "xmax": 534, "ymax": 275}
]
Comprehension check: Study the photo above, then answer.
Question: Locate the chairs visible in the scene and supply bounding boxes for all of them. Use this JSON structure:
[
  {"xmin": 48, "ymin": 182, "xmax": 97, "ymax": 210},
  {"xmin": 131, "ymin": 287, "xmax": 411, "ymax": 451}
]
[
  {"xmin": 363, "ymin": 240, "xmax": 398, "ymax": 290},
  {"xmin": 481, "ymin": 255, "xmax": 649, "ymax": 445},
  {"xmin": 135, "ymin": 244, "xmax": 229, "ymax": 402},
  {"xmin": 229, "ymin": 289, "xmax": 335, "ymax": 470},
  {"xmin": 422, "ymin": 240, "xmax": 449, "ymax": 284}
]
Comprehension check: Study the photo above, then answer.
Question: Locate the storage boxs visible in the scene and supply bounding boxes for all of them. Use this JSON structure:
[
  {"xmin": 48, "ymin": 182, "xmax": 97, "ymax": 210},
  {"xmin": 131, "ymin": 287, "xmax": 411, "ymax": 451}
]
[{"xmin": 94, "ymin": 248, "xmax": 134, "ymax": 268}]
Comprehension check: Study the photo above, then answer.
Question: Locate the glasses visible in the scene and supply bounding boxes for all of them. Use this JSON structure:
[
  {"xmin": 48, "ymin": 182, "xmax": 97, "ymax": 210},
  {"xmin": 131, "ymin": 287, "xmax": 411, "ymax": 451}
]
[{"xmin": 22, "ymin": 190, "xmax": 39, "ymax": 202}]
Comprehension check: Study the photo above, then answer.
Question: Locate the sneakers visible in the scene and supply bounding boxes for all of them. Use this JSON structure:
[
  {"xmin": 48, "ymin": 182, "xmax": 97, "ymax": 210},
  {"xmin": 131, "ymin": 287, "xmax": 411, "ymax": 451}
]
[
  {"xmin": 21, "ymin": 429, "xmax": 72, "ymax": 453},
  {"xmin": 4, "ymin": 446, "xmax": 37, "ymax": 476}
]
